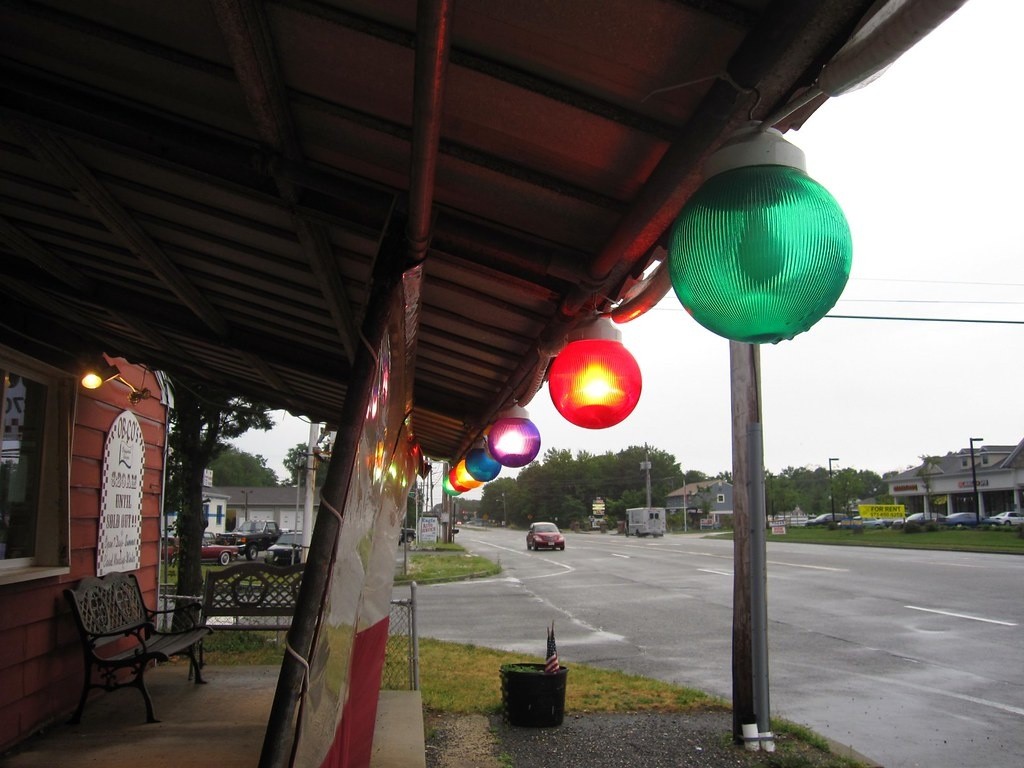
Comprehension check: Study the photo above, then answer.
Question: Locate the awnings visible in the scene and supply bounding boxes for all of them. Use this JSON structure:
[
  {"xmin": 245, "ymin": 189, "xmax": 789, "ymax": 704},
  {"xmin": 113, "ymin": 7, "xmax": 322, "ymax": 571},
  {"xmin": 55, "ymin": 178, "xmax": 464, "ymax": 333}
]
[{"xmin": 0, "ymin": 0, "xmax": 875, "ymax": 469}]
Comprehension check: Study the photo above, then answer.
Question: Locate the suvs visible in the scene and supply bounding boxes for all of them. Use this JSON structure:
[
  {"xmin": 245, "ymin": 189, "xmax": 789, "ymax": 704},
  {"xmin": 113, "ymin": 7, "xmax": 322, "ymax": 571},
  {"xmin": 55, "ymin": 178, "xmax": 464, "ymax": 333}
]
[{"xmin": 215, "ymin": 520, "xmax": 283, "ymax": 561}]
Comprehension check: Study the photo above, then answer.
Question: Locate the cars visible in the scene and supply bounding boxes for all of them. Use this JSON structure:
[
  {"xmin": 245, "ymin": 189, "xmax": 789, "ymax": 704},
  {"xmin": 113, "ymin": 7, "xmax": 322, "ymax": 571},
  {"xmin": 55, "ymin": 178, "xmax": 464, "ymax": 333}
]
[
  {"xmin": 267, "ymin": 531, "xmax": 304, "ymax": 567},
  {"xmin": 837, "ymin": 516, "xmax": 894, "ymax": 528},
  {"xmin": 892, "ymin": 512, "xmax": 947, "ymax": 528},
  {"xmin": 805, "ymin": 513, "xmax": 849, "ymax": 526},
  {"xmin": 526, "ymin": 521, "xmax": 564, "ymax": 554},
  {"xmin": 161, "ymin": 535, "xmax": 238, "ymax": 567},
  {"xmin": 989, "ymin": 512, "xmax": 1024, "ymax": 526},
  {"xmin": 936, "ymin": 511, "xmax": 1001, "ymax": 528}
]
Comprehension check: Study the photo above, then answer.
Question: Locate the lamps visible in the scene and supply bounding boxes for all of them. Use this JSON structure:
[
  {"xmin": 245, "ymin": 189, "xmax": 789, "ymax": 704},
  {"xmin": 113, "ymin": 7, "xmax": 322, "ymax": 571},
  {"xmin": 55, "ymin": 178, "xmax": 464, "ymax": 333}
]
[
  {"xmin": 488, "ymin": 400, "xmax": 540, "ymax": 468},
  {"xmin": 129, "ymin": 387, "xmax": 152, "ymax": 405},
  {"xmin": 81, "ymin": 356, "xmax": 122, "ymax": 388},
  {"xmin": 442, "ymin": 441, "xmax": 501, "ymax": 496},
  {"xmin": 549, "ymin": 317, "xmax": 642, "ymax": 429},
  {"xmin": 668, "ymin": 120, "xmax": 851, "ymax": 346}
]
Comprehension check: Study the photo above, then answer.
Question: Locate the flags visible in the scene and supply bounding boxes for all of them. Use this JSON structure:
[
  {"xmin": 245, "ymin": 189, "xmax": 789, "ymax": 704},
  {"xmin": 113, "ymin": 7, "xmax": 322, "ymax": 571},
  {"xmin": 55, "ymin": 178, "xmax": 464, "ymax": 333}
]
[{"xmin": 544, "ymin": 620, "xmax": 560, "ymax": 672}]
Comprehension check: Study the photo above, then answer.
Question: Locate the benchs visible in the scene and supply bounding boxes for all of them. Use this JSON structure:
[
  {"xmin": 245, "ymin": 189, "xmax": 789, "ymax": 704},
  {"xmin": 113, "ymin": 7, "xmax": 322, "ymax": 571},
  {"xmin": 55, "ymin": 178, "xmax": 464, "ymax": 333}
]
[
  {"xmin": 62, "ymin": 572, "xmax": 214, "ymax": 723},
  {"xmin": 187, "ymin": 563, "xmax": 307, "ymax": 680}
]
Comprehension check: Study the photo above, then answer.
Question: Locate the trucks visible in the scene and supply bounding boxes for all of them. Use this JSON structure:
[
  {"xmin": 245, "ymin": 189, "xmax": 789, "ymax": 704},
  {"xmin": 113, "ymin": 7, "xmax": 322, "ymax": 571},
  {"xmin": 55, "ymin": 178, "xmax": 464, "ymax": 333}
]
[{"xmin": 624, "ymin": 507, "xmax": 666, "ymax": 538}]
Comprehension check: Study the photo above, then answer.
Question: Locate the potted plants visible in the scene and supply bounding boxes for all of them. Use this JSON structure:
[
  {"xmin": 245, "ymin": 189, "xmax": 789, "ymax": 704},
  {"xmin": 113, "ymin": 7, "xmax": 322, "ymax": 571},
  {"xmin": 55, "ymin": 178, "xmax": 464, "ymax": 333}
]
[{"xmin": 499, "ymin": 662, "xmax": 569, "ymax": 725}]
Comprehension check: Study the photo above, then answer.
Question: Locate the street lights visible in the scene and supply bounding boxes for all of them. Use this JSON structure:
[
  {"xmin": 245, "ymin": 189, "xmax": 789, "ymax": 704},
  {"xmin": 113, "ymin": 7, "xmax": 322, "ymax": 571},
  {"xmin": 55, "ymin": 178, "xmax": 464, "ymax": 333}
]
[
  {"xmin": 770, "ymin": 475, "xmax": 780, "ymax": 521},
  {"xmin": 496, "ymin": 493, "xmax": 506, "ymax": 525},
  {"xmin": 969, "ymin": 437, "xmax": 984, "ymax": 526},
  {"xmin": 829, "ymin": 458, "xmax": 839, "ymax": 524}
]
